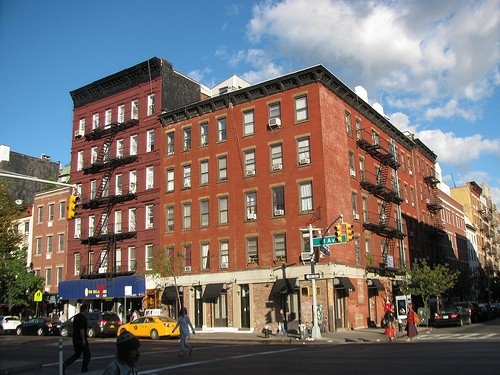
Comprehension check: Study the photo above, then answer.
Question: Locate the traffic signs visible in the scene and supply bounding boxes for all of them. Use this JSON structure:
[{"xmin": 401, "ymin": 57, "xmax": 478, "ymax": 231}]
[{"xmin": 304, "ymin": 274, "xmax": 320, "ymax": 280}]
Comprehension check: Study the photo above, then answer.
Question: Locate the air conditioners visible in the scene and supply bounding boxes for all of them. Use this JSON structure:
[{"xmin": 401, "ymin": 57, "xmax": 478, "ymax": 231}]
[
  {"xmin": 247, "ymin": 214, "xmax": 257, "ymax": 220},
  {"xmin": 301, "ymin": 252, "xmax": 310, "ymax": 260},
  {"xmin": 268, "ymin": 118, "xmax": 281, "ymax": 131},
  {"xmin": 184, "ymin": 266, "xmax": 191, "ymax": 271},
  {"xmin": 274, "ymin": 210, "xmax": 285, "ymax": 216},
  {"xmin": 353, "ymin": 214, "xmax": 359, "ymax": 220},
  {"xmin": 350, "ymin": 170, "xmax": 355, "ymax": 176},
  {"xmin": 220, "ymin": 263, "xmax": 229, "ymax": 269}
]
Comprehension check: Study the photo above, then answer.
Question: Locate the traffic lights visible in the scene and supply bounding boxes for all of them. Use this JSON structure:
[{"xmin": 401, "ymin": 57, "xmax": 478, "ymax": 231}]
[
  {"xmin": 68, "ymin": 195, "xmax": 80, "ymax": 220},
  {"xmin": 345, "ymin": 224, "xmax": 354, "ymax": 242},
  {"xmin": 334, "ymin": 225, "xmax": 344, "ymax": 242}
]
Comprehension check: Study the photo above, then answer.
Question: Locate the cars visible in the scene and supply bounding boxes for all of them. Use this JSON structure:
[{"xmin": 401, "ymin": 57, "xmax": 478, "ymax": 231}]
[
  {"xmin": 58, "ymin": 311, "xmax": 122, "ymax": 338},
  {"xmin": 116, "ymin": 316, "xmax": 183, "ymax": 341},
  {"xmin": 16, "ymin": 318, "xmax": 62, "ymax": 337},
  {"xmin": 434, "ymin": 307, "xmax": 473, "ymax": 326},
  {"xmin": 0, "ymin": 315, "xmax": 23, "ymax": 333},
  {"xmin": 456, "ymin": 302, "xmax": 500, "ymax": 324}
]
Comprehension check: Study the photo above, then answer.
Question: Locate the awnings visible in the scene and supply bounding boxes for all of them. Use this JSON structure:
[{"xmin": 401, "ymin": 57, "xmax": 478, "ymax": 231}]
[
  {"xmin": 336, "ymin": 277, "xmax": 355, "ymax": 292},
  {"xmin": 203, "ymin": 283, "xmax": 224, "ymax": 303},
  {"xmin": 370, "ymin": 280, "xmax": 385, "ymax": 290},
  {"xmin": 269, "ymin": 278, "xmax": 296, "ymax": 300},
  {"xmin": 161, "ymin": 286, "xmax": 180, "ymax": 304}
]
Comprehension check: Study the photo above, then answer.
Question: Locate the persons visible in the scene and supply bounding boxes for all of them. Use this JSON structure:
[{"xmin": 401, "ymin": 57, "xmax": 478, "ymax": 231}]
[
  {"xmin": 384, "ymin": 305, "xmax": 397, "ymax": 342},
  {"xmin": 172, "ymin": 308, "xmax": 196, "ymax": 356},
  {"xmin": 406, "ymin": 305, "xmax": 419, "ymax": 342},
  {"xmin": 63, "ymin": 304, "xmax": 91, "ymax": 375},
  {"xmin": 277, "ymin": 309, "xmax": 287, "ymax": 336},
  {"xmin": 104, "ymin": 331, "xmax": 141, "ymax": 375}
]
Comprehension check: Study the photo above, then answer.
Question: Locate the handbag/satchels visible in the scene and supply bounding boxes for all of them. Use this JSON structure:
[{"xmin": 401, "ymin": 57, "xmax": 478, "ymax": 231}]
[{"xmin": 381, "ymin": 316, "xmax": 389, "ymax": 328}]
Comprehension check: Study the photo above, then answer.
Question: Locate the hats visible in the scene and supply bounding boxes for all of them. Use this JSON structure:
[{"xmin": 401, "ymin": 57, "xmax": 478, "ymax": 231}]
[{"xmin": 117, "ymin": 330, "xmax": 141, "ymax": 354}]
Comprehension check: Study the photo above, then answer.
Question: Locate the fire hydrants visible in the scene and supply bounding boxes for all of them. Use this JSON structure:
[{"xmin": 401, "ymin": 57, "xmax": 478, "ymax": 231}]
[
  {"xmin": 398, "ymin": 319, "xmax": 403, "ymax": 332},
  {"xmin": 262, "ymin": 326, "xmax": 272, "ymax": 338}
]
